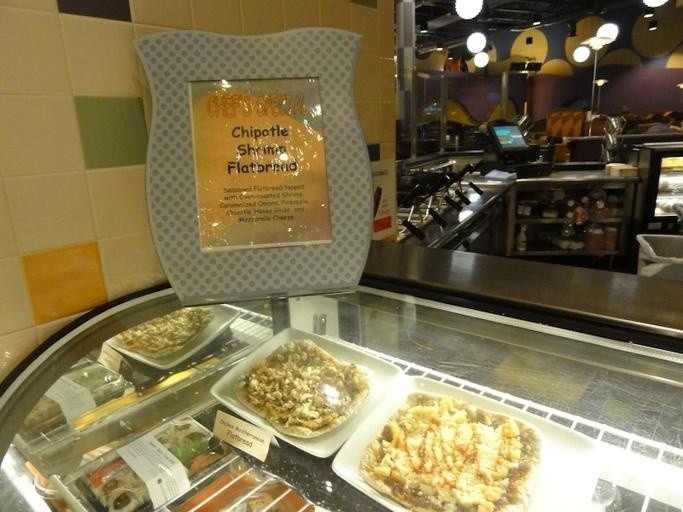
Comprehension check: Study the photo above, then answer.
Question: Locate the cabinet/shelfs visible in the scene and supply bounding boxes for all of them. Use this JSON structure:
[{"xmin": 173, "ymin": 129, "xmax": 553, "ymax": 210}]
[
  {"xmin": 2, "ymin": 241, "xmax": 682, "ymax": 511},
  {"xmin": 501, "ymin": 169, "xmax": 640, "ymax": 274}
]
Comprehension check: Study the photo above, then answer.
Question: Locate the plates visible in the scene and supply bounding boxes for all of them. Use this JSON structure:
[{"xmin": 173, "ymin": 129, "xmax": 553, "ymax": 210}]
[
  {"xmin": 210, "ymin": 326, "xmax": 403, "ymax": 458},
  {"xmin": 331, "ymin": 378, "xmax": 603, "ymax": 510},
  {"xmin": 105, "ymin": 304, "xmax": 243, "ymax": 372}
]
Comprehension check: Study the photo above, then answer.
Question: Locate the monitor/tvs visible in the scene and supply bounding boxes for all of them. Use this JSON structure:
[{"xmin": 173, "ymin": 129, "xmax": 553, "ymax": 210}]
[{"xmin": 487, "ymin": 120, "xmax": 530, "ymax": 152}]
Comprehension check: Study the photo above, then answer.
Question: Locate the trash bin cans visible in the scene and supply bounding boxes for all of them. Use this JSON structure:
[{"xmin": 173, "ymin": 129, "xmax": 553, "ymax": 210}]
[{"xmin": 635, "ymin": 234, "xmax": 682, "ymax": 281}]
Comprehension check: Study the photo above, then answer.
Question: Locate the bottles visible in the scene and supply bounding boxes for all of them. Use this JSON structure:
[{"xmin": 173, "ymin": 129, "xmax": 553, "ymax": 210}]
[
  {"xmin": 517, "ymin": 226, "xmax": 527, "ymax": 252},
  {"xmin": 560, "ymin": 198, "xmax": 585, "ymax": 250}
]
